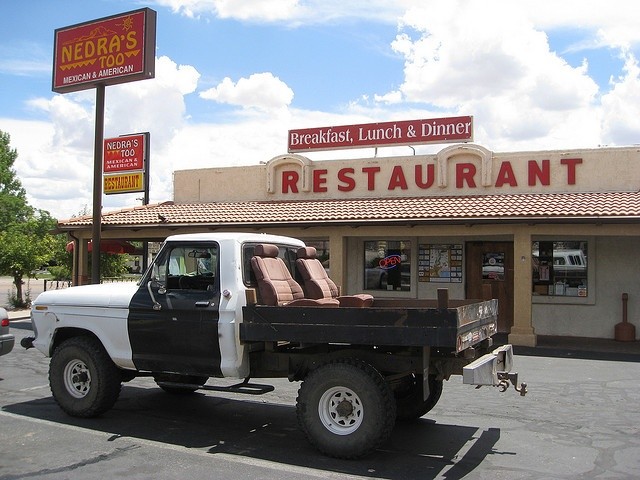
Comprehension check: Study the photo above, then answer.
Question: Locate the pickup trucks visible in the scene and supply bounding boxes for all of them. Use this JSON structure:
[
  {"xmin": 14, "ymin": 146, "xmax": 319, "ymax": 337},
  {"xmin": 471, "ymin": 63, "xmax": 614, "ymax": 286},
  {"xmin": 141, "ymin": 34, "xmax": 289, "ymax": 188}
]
[{"xmin": 21, "ymin": 232, "xmax": 527, "ymax": 461}]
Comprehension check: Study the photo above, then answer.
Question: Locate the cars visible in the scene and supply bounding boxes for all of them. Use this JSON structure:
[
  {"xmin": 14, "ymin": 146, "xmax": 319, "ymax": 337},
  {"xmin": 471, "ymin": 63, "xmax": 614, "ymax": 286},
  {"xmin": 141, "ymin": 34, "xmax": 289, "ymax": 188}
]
[
  {"xmin": 0, "ymin": 307, "xmax": 15, "ymax": 356},
  {"xmin": 322, "ymin": 251, "xmax": 387, "ymax": 290}
]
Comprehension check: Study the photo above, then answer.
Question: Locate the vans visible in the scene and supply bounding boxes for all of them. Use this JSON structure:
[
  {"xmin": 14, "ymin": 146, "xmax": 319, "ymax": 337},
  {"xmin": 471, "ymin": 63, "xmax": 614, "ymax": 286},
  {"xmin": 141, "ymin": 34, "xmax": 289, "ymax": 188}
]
[{"xmin": 532, "ymin": 249, "xmax": 586, "ymax": 270}]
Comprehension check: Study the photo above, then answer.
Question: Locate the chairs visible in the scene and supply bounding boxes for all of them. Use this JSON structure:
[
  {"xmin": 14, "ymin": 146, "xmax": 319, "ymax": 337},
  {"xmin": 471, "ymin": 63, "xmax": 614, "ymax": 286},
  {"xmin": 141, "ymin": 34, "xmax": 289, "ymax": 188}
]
[
  {"xmin": 295, "ymin": 247, "xmax": 375, "ymax": 308},
  {"xmin": 283, "ymin": 249, "xmax": 298, "ymax": 280},
  {"xmin": 251, "ymin": 244, "xmax": 340, "ymax": 308}
]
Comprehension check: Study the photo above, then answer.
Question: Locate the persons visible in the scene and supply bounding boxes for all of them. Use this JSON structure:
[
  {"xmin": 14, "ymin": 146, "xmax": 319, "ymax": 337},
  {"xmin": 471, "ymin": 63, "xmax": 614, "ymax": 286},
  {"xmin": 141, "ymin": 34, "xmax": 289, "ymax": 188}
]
[
  {"xmin": 369, "ymin": 249, "xmax": 388, "ymax": 268},
  {"xmin": 401, "ymin": 242, "xmax": 407, "ymax": 255},
  {"xmin": 136, "ymin": 266, "xmax": 141, "ymax": 274}
]
